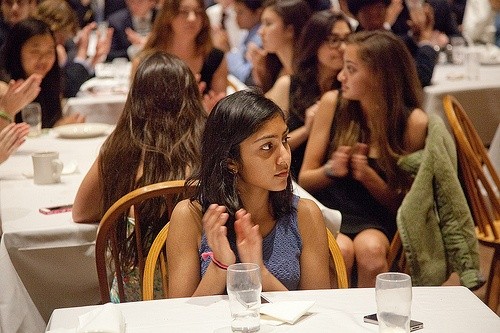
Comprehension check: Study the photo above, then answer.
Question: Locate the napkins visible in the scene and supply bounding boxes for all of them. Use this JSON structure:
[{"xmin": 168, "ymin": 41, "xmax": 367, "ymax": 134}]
[{"xmin": 259, "ymin": 303, "xmax": 313, "ymax": 325}]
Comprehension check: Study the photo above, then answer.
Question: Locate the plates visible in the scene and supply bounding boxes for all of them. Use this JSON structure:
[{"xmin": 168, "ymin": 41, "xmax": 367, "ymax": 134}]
[{"xmin": 55, "ymin": 122, "xmax": 111, "ymax": 138}]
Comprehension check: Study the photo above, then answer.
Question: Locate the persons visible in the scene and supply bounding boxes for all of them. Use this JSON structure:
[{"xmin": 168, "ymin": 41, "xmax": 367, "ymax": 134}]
[{"xmin": 0, "ymin": 0, "xmax": 500, "ymax": 305}]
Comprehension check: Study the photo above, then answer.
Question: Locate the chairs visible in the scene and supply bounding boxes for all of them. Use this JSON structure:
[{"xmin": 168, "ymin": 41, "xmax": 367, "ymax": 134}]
[
  {"xmin": 442, "ymin": 94, "xmax": 499, "ymax": 311},
  {"xmin": 143, "ymin": 220, "xmax": 349, "ymax": 300},
  {"xmin": 387, "ymin": 114, "xmax": 486, "ymax": 292},
  {"xmin": 95, "ymin": 180, "xmax": 202, "ymax": 304}
]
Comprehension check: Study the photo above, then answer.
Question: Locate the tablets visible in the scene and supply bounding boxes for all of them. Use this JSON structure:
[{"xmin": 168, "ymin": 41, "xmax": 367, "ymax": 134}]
[{"xmin": 86, "ymin": 20, "xmax": 109, "ymax": 57}]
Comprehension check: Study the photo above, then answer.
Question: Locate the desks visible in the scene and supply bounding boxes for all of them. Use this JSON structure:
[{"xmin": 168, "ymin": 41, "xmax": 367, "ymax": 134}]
[
  {"xmin": 0, "ymin": 60, "xmax": 133, "ymax": 324},
  {"xmin": 46, "ymin": 286, "xmax": 500, "ymax": 333},
  {"xmin": 423, "ymin": 61, "xmax": 500, "ymax": 146}
]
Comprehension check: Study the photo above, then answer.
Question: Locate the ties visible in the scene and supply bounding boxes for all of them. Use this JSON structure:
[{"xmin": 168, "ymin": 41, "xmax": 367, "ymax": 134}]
[{"xmin": 220, "ymin": 11, "xmax": 225, "ymax": 32}]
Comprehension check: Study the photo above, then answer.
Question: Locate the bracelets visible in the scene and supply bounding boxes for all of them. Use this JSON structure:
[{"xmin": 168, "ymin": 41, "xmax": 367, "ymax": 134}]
[
  {"xmin": 201, "ymin": 252, "xmax": 229, "ymax": 270},
  {"xmin": 324, "ymin": 161, "xmax": 337, "ymax": 180},
  {"xmin": 0, "ymin": 111, "xmax": 14, "ymax": 123}
]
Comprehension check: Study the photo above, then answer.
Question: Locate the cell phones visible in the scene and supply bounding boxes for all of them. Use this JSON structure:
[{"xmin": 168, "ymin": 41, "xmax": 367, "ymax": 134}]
[
  {"xmin": 363, "ymin": 311, "xmax": 423, "ymax": 333},
  {"xmin": 39, "ymin": 203, "xmax": 74, "ymax": 215},
  {"xmin": 237, "ymin": 290, "xmax": 272, "ymax": 309}
]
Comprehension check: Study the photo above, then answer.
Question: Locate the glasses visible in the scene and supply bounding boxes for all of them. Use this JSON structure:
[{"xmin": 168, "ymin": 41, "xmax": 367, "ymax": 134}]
[{"xmin": 325, "ymin": 33, "xmax": 352, "ymax": 48}]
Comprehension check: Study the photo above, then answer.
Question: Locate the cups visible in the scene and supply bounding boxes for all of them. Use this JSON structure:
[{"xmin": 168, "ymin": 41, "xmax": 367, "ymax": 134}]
[
  {"xmin": 451, "ymin": 36, "xmax": 465, "ymax": 63},
  {"xmin": 374, "ymin": 272, "xmax": 412, "ymax": 333},
  {"xmin": 32, "ymin": 150, "xmax": 64, "ymax": 185},
  {"xmin": 464, "ymin": 52, "xmax": 481, "ymax": 81},
  {"xmin": 20, "ymin": 101, "xmax": 42, "ymax": 139},
  {"xmin": 227, "ymin": 262, "xmax": 261, "ymax": 333}
]
